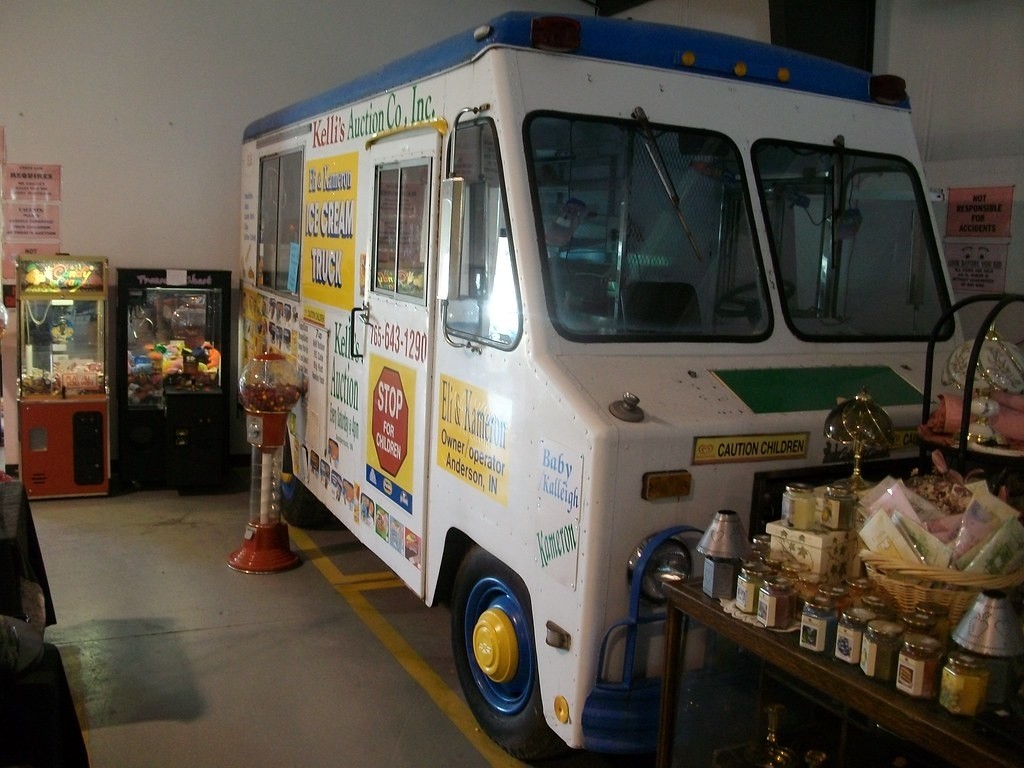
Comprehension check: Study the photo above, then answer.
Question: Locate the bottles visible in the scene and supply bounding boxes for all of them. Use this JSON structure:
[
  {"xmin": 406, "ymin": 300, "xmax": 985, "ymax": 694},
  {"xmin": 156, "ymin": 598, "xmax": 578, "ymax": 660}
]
[
  {"xmin": 735, "ymin": 534, "xmax": 990, "ymax": 717},
  {"xmin": 820, "ymin": 484, "xmax": 855, "ymax": 531},
  {"xmin": 239, "ymin": 352, "xmax": 301, "ymax": 412},
  {"xmin": 782, "ymin": 483, "xmax": 816, "ymax": 529}
]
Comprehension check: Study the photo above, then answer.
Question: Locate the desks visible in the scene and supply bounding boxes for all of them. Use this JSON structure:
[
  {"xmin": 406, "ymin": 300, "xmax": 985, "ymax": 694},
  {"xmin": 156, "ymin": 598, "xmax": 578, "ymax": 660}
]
[
  {"xmin": 0, "ymin": 482, "xmax": 56, "ymax": 629},
  {"xmin": 0, "ymin": 642, "xmax": 90, "ymax": 768}
]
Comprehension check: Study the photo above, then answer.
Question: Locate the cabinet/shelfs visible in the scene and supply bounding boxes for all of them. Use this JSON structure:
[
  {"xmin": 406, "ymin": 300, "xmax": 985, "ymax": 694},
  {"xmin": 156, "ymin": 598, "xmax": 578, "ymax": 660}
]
[
  {"xmin": 918, "ymin": 291, "xmax": 1024, "ymax": 478},
  {"xmin": 656, "ymin": 575, "xmax": 1022, "ymax": 768}
]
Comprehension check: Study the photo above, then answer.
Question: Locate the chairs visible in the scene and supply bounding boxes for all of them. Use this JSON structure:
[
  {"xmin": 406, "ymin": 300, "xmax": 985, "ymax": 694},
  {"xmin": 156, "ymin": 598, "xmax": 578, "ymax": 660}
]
[{"xmin": 621, "ymin": 280, "xmax": 704, "ymax": 329}]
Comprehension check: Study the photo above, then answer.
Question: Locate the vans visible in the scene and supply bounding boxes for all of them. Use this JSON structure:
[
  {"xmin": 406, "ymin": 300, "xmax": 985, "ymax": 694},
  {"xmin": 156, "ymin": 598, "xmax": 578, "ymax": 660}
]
[{"xmin": 238, "ymin": 9, "xmax": 968, "ymax": 768}]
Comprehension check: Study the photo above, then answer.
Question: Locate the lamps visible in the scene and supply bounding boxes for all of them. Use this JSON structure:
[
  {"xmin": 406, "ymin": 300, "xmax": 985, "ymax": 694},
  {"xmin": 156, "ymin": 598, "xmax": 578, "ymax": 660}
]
[
  {"xmin": 939, "ymin": 320, "xmax": 1024, "ymax": 448},
  {"xmin": 822, "ymin": 385, "xmax": 896, "ymax": 498},
  {"xmin": 697, "ymin": 509, "xmax": 751, "ymax": 598},
  {"xmin": 952, "ymin": 588, "xmax": 1024, "ymax": 705}
]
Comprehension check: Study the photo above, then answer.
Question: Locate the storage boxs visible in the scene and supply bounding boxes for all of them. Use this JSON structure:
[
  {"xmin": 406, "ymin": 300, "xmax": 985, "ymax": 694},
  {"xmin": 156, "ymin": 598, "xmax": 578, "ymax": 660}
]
[{"xmin": 765, "ymin": 485, "xmax": 861, "ymax": 585}]
